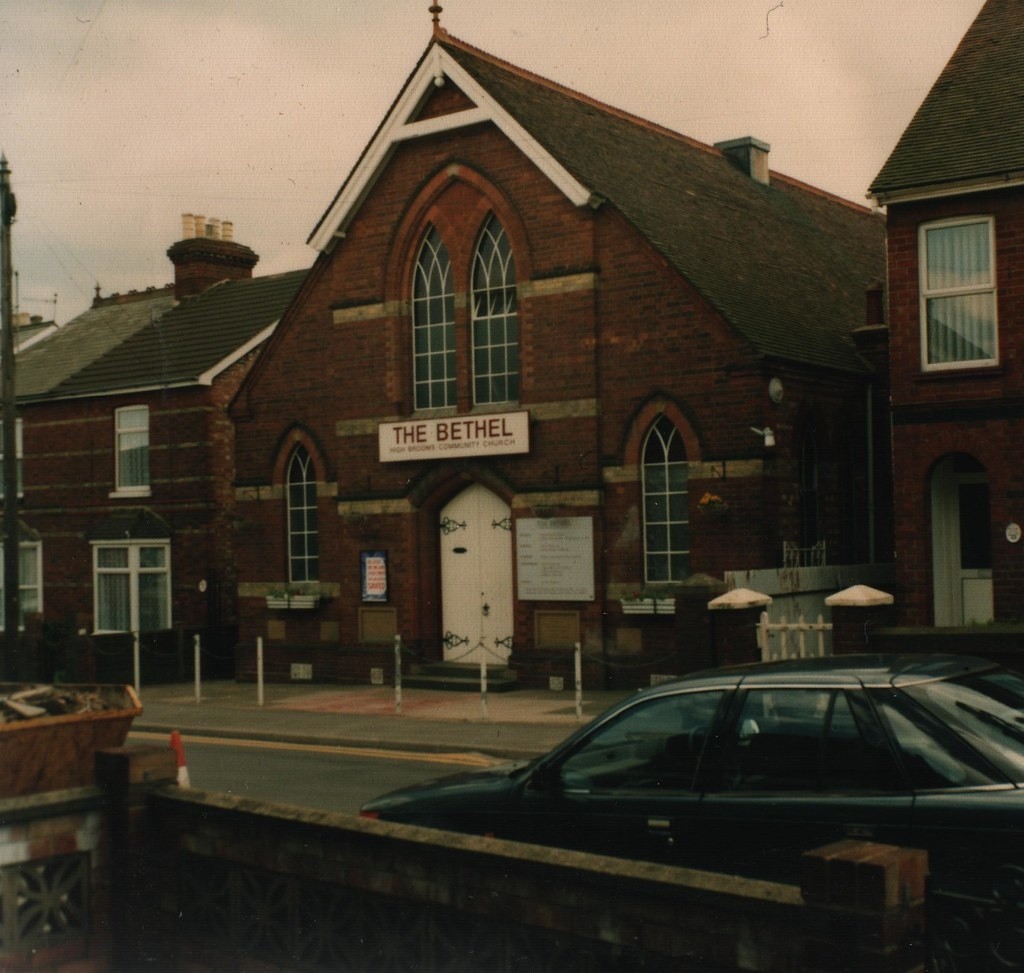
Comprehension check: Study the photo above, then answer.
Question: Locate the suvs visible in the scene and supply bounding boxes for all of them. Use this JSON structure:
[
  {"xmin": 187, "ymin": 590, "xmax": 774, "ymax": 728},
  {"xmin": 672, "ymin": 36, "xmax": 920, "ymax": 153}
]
[{"xmin": 352, "ymin": 649, "xmax": 1023, "ymax": 972}]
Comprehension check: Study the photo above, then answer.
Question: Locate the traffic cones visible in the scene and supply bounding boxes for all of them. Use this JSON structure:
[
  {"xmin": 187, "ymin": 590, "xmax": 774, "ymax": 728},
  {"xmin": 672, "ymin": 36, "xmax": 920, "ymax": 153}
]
[{"xmin": 166, "ymin": 726, "xmax": 191, "ymax": 788}]
